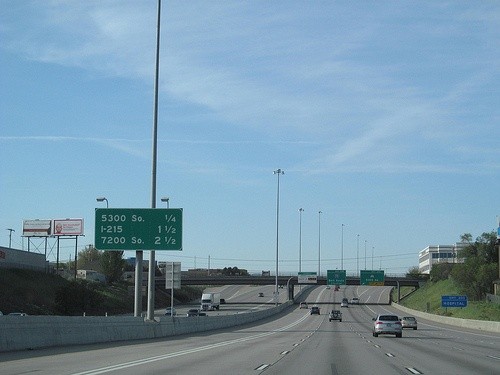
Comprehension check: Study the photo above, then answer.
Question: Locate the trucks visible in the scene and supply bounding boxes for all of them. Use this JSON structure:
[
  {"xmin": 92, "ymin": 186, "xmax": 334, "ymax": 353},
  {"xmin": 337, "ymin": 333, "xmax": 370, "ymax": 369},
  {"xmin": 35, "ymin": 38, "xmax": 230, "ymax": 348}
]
[
  {"xmin": 200, "ymin": 292, "xmax": 220, "ymax": 311},
  {"xmin": 186, "ymin": 309, "xmax": 206, "ymax": 317}
]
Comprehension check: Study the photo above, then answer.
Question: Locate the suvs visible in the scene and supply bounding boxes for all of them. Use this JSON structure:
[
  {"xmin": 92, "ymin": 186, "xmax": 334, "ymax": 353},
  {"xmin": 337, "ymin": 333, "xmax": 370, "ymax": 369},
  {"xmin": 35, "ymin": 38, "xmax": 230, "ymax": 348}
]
[
  {"xmin": 372, "ymin": 313, "xmax": 403, "ymax": 338},
  {"xmin": 328, "ymin": 309, "xmax": 342, "ymax": 322}
]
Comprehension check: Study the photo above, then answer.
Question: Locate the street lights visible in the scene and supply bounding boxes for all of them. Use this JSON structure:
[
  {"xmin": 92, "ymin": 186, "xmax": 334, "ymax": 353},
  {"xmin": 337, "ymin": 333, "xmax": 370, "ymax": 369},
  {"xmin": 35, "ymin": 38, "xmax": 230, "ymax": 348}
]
[
  {"xmin": 273, "ymin": 168, "xmax": 285, "ymax": 306},
  {"xmin": 317, "ymin": 210, "xmax": 322, "ymax": 276},
  {"xmin": 341, "ymin": 223, "xmax": 343, "ymax": 270},
  {"xmin": 364, "ymin": 239, "xmax": 368, "ymax": 271},
  {"xmin": 372, "ymin": 246, "xmax": 374, "ymax": 270},
  {"xmin": 357, "ymin": 234, "xmax": 360, "ymax": 278},
  {"xmin": 298, "ymin": 208, "xmax": 305, "ymax": 291}
]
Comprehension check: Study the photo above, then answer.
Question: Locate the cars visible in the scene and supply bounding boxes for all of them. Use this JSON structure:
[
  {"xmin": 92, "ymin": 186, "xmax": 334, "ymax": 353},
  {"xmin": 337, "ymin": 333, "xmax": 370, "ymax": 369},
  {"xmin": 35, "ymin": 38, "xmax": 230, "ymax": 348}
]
[
  {"xmin": 219, "ymin": 298, "xmax": 225, "ymax": 304},
  {"xmin": 401, "ymin": 316, "xmax": 417, "ymax": 330},
  {"xmin": 258, "ymin": 293, "xmax": 264, "ymax": 297},
  {"xmin": 310, "ymin": 306, "xmax": 321, "ymax": 315},
  {"xmin": 326, "ymin": 285, "xmax": 340, "ymax": 291},
  {"xmin": 8, "ymin": 312, "xmax": 28, "ymax": 316},
  {"xmin": 300, "ymin": 302, "xmax": 308, "ymax": 309},
  {"xmin": 340, "ymin": 297, "xmax": 349, "ymax": 308},
  {"xmin": 165, "ymin": 307, "xmax": 177, "ymax": 317},
  {"xmin": 350, "ymin": 297, "xmax": 360, "ymax": 304}
]
